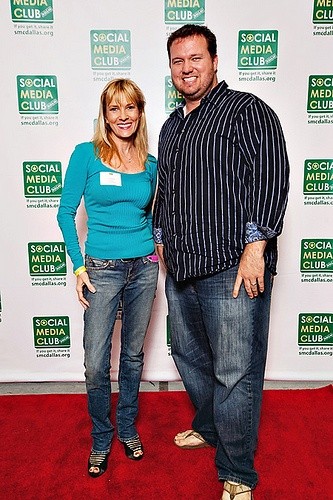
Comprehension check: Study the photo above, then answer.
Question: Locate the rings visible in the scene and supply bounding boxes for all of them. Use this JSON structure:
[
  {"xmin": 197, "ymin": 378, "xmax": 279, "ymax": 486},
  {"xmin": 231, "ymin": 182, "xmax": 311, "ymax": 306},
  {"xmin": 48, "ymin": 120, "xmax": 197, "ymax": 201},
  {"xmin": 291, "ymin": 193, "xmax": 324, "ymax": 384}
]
[{"xmin": 251, "ymin": 283, "xmax": 257, "ymax": 286}]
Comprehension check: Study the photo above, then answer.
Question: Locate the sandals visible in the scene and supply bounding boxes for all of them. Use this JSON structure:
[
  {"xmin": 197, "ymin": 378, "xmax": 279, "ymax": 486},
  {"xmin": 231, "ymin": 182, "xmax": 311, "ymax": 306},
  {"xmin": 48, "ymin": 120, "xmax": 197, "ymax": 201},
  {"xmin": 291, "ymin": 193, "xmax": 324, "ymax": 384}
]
[
  {"xmin": 118, "ymin": 434, "xmax": 143, "ymax": 461},
  {"xmin": 88, "ymin": 448, "xmax": 110, "ymax": 477}
]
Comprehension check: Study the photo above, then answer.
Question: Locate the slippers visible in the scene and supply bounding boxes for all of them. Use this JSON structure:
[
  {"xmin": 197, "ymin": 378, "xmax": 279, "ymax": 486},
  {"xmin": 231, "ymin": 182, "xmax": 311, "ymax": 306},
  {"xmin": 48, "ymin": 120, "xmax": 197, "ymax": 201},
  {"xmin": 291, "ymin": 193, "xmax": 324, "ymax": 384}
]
[
  {"xmin": 221, "ymin": 481, "xmax": 254, "ymax": 500},
  {"xmin": 174, "ymin": 429, "xmax": 211, "ymax": 448}
]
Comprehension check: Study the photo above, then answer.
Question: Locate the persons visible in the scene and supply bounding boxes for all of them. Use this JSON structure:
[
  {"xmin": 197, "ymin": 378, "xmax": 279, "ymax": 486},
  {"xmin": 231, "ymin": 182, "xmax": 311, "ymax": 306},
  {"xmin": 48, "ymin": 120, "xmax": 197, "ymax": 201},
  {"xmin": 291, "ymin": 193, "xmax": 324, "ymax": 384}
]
[
  {"xmin": 152, "ymin": 24, "xmax": 290, "ymax": 500},
  {"xmin": 56, "ymin": 79, "xmax": 157, "ymax": 477}
]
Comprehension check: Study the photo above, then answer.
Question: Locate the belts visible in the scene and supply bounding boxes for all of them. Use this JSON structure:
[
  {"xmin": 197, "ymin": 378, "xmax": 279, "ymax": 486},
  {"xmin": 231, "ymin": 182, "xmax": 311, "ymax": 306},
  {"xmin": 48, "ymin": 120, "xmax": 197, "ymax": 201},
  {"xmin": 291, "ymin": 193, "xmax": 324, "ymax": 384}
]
[{"xmin": 121, "ymin": 257, "xmax": 142, "ymax": 263}]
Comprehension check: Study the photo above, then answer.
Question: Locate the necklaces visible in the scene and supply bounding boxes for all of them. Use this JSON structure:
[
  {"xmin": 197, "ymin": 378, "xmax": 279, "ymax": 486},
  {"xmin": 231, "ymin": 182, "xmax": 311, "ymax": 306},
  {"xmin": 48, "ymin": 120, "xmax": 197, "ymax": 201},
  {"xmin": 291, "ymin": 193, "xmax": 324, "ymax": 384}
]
[{"xmin": 128, "ymin": 159, "xmax": 131, "ymax": 163}]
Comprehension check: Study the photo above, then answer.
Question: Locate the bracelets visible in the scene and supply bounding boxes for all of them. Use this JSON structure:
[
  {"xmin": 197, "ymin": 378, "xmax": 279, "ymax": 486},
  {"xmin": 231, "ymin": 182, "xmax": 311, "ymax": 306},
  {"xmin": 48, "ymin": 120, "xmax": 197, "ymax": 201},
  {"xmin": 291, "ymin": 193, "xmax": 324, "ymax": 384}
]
[{"xmin": 75, "ymin": 266, "xmax": 87, "ymax": 277}]
